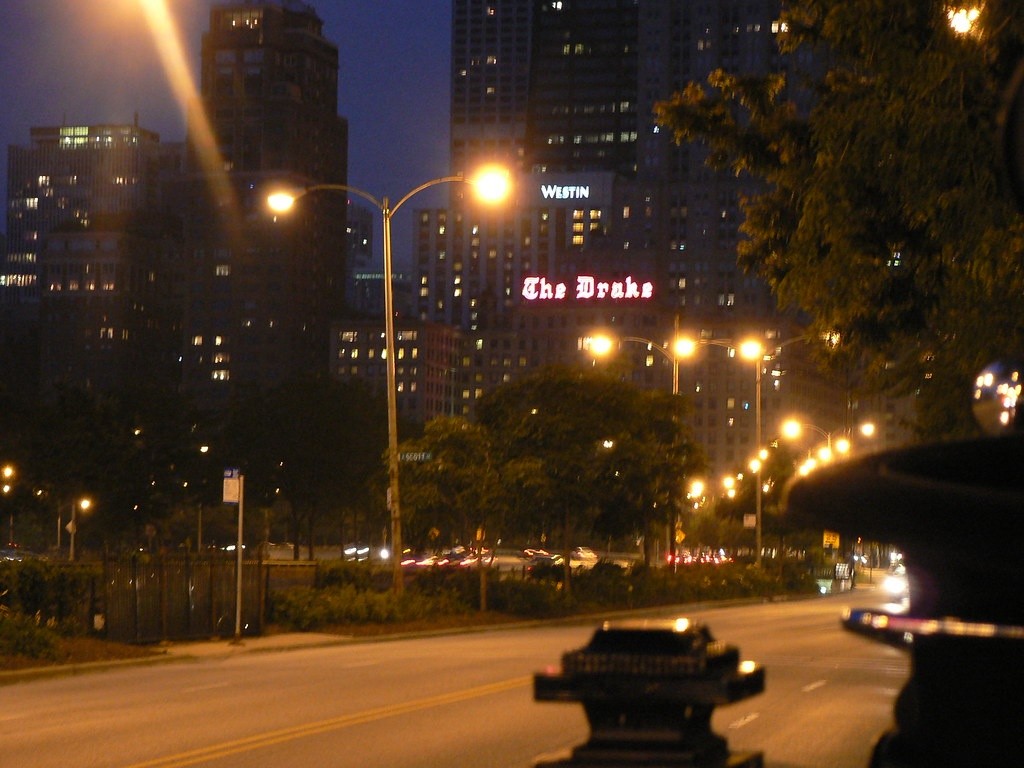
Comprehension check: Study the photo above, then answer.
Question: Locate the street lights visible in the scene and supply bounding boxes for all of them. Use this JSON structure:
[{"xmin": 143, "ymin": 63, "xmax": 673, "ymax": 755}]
[
  {"xmin": 576, "ymin": 315, "xmax": 763, "ymax": 579},
  {"xmin": 69, "ymin": 495, "xmax": 95, "ymax": 560},
  {"xmin": 674, "ymin": 325, "xmax": 844, "ymax": 565},
  {"xmin": 778, "ymin": 417, "xmax": 876, "ymax": 464},
  {"xmin": 261, "ymin": 162, "xmax": 510, "ymax": 606}
]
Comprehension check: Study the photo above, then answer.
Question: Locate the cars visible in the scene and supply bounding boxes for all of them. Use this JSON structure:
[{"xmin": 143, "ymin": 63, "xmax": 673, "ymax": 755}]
[{"xmin": 523, "ymin": 546, "xmax": 597, "ymax": 569}]
